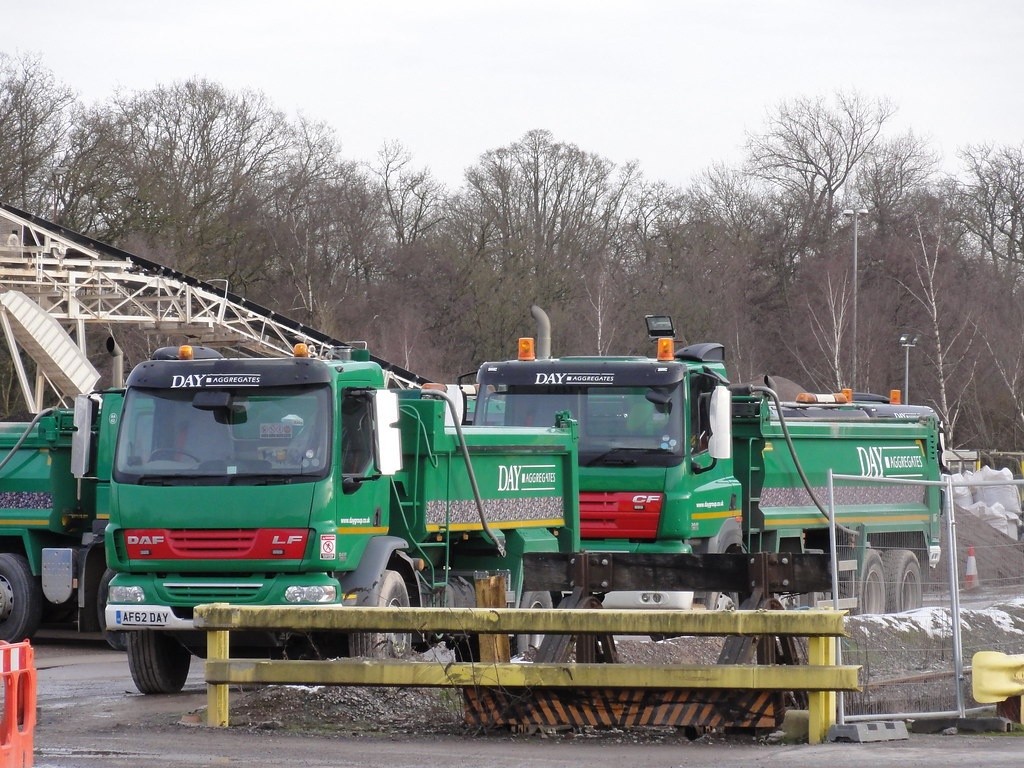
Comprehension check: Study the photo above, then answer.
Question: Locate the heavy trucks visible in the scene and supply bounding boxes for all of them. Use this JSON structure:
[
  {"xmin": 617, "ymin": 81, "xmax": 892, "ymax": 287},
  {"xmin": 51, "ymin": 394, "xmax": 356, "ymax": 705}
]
[
  {"xmin": 69, "ymin": 330, "xmax": 584, "ymax": 699},
  {"xmin": 468, "ymin": 303, "xmax": 947, "ymax": 617},
  {"xmin": 0, "ymin": 403, "xmax": 92, "ymax": 645}
]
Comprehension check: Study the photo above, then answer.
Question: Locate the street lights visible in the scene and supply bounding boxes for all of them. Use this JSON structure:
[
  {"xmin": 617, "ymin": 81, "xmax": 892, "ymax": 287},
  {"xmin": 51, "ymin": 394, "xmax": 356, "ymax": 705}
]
[
  {"xmin": 842, "ymin": 208, "xmax": 871, "ymax": 389},
  {"xmin": 899, "ymin": 333, "xmax": 920, "ymax": 406}
]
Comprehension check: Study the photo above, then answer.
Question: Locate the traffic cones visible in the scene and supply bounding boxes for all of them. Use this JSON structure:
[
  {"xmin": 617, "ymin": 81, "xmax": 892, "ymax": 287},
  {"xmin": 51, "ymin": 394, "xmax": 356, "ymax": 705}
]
[{"xmin": 964, "ymin": 543, "xmax": 982, "ymax": 595}]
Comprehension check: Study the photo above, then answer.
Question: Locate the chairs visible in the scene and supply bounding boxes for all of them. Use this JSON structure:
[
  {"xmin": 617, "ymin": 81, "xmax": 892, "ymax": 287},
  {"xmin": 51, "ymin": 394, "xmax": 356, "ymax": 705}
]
[{"xmin": 186, "ymin": 417, "xmax": 233, "ymax": 462}]
